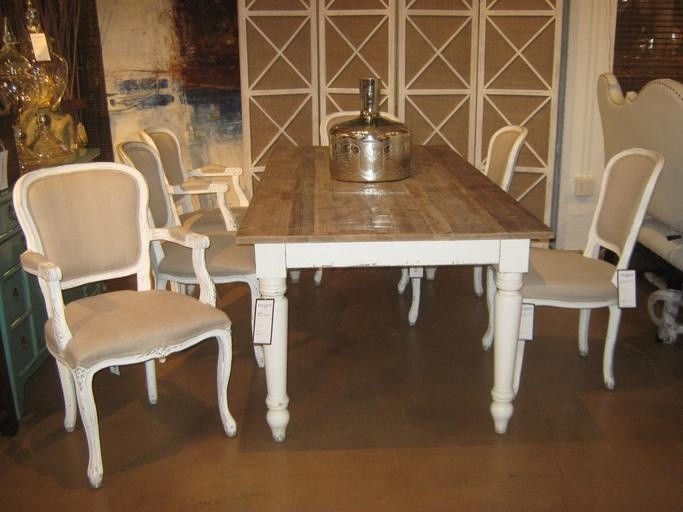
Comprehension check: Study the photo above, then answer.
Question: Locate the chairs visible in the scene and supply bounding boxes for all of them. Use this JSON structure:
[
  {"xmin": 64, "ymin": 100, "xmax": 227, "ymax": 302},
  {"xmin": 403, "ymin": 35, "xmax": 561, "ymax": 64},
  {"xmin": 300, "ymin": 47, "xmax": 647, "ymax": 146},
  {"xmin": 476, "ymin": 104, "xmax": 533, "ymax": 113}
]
[
  {"xmin": 13, "ymin": 162, "xmax": 237, "ymax": 487},
  {"xmin": 136, "ymin": 127, "xmax": 249, "ymax": 234},
  {"xmin": 399, "ymin": 126, "xmax": 530, "ymax": 326},
  {"xmin": 481, "ymin": 148, "xmax": 664, "ymax": 401},
  {"xmin": 596, "ymin": 70, "xmax": 682, "ymax": 280},
  {"xmin": 116, "ymin": 140, "xmax": 265, "ymax": 370},
  {"xmin": 312, "ymin": 111, "xmax": 402, "ymax": 286}
]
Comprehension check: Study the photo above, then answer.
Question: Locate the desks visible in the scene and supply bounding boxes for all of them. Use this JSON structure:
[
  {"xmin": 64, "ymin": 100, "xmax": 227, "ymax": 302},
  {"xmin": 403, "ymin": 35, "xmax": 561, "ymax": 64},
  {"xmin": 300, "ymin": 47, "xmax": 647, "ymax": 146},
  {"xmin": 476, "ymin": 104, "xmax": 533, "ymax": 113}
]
[{"xmin": 234, "ymin": 141, "xmax": 556, "ymax": 441}]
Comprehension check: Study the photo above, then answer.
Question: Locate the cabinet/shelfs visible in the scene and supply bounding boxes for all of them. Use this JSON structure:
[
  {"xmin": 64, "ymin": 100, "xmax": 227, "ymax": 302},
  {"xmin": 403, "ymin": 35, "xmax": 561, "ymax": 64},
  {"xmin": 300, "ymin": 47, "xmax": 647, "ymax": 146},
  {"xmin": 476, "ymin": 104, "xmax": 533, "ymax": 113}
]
[{"xmin": 0, "ymin": 145, "xmax": 107, "ymax": 422}]
